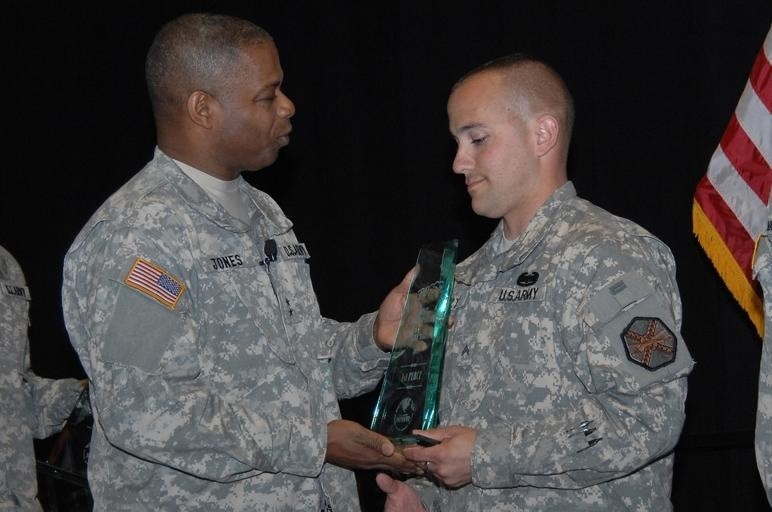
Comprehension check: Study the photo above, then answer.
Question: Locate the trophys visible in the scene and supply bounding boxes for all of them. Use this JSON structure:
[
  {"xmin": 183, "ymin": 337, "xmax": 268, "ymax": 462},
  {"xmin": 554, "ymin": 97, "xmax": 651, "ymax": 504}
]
[{"xmin": 368, "ymin": 240, "xmax": 461, "ymax": 447}]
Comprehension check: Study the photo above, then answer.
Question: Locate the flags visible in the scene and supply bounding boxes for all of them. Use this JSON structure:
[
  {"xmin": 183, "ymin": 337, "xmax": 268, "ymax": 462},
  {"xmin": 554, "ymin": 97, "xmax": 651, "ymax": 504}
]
[{"xmin": 692, "ymin": 26, "xmax": 772, "ymax": 340}]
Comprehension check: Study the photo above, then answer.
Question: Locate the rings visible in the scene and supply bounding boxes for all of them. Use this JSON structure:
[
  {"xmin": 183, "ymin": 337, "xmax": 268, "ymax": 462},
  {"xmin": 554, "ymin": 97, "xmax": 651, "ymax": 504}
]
[{"xmin": 425, "ymin": 461, "xmax": 429, "ymax": 473}]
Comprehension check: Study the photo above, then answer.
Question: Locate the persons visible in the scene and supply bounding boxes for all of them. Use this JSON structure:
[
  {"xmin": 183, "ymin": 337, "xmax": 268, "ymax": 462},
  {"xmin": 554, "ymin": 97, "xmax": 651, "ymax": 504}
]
[
  {"xmin": 61, "ymin": 13, "xmax": 455, "ymax": 511},
  {"xmin": 754, "ymin": 227, "xmax": 772, "ymax": 509},
  {"xmin": 0, "ymin": 247, "xmax": 88, "ymax": 512},
  {"xmin": 375, "ymin": 52, "xmax": 698, "ymax": 511}
]
